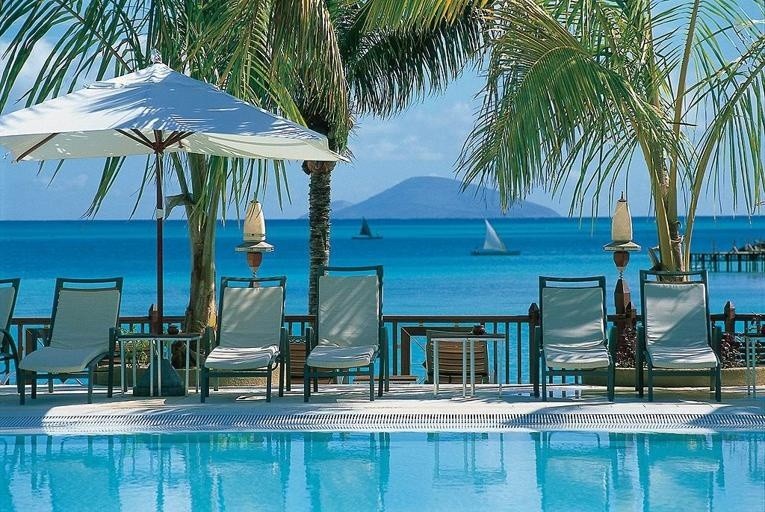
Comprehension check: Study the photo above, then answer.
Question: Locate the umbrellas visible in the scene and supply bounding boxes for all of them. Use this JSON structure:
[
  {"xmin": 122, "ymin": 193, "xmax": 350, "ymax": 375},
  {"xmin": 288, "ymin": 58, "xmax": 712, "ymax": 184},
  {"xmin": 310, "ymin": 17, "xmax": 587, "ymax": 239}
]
[{"xmin": 0, "ymin": 50, "xmax": 349, "ymax": 358}]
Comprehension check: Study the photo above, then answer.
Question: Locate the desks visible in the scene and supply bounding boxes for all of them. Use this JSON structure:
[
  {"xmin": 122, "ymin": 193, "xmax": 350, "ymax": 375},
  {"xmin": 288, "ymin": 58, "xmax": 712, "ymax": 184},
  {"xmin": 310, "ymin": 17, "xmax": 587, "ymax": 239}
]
[
  {"xmin": 428, "ymin": 333, "xmax": 507, "ymax": 400},
  {"xmin": 116, "ymin": 332, "xmax": 202, "ymax": 399}
]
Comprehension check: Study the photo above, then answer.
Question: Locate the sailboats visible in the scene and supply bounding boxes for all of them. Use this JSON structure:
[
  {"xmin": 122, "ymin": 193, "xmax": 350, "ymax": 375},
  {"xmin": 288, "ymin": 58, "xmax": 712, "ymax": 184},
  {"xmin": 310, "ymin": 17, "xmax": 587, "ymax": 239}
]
[
  {"xmin": 351, "ymin": 216, "xmax": 382, "ymax": 239},
  {"xmin": 470, "ymin": 218, "xmax": 521, "ymax": 255}
]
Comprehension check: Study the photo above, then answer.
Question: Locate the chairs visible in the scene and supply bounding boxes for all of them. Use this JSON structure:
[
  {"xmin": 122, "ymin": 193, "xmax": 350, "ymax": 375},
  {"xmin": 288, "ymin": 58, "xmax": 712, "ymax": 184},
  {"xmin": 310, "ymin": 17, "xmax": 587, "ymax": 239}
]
[
  {"xmin": 634, "ymin": 270, "xmax": 721, "ymax": 402},
  {"xmin": 0, "ymin": 265, "xmax": 723, "ymax": 407},
  {"xmin": 303, "ymin": 266, "xmax": 389, "ymax": 403},
  {"xmin": 0, "ymin": 278, "xmax": 26, "ymax": 401},
  {"xmin": 201, "ymin": 276, "xmax": 291, "ymax": 404},
  {"xmin": 530, "ymin": 276, "xmax": 615, "ymax": 401},
  {"xmin": 19, "ymin": 277, "xmax": 124, "ymax": 403}
]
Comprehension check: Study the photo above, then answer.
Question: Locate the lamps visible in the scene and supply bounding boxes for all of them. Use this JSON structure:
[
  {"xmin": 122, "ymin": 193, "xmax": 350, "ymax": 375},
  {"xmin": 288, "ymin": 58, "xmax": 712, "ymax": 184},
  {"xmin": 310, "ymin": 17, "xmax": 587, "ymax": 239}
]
[
  {"xmin": 603, "ymin": 191, "xmax": 640, "ymax": 277},
  {"xmin": 237, "ymin": 192, "xmax": 273, "ymax": 277}
]
